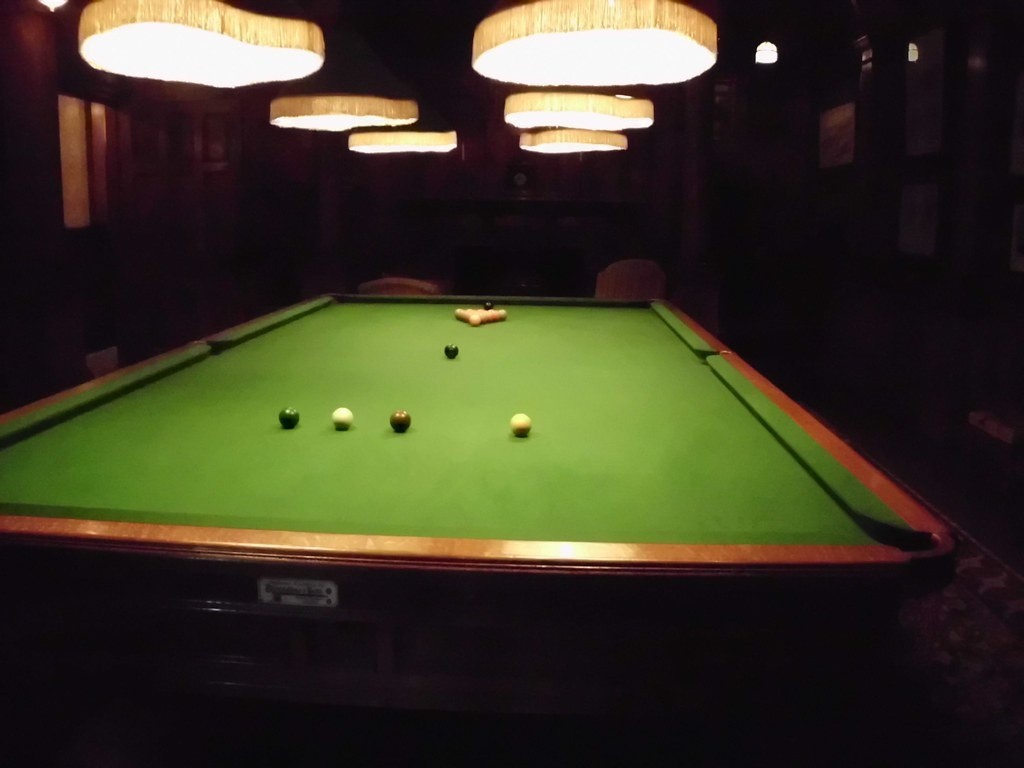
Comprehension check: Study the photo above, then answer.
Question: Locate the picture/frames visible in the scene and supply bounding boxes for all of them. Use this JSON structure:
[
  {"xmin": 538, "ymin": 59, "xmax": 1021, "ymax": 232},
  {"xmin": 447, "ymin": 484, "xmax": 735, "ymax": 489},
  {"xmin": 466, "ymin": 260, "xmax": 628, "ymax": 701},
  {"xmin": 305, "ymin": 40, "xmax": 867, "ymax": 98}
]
[
  {"xmin": 894, "ymin": 183, "xmax": 945, "ymax": 259},
  {"xmin": 1004, "ymin": 78, "xmax": 1024, "ymax": 182},
  {"xmin": 814, "ymin": 100, "xmax": 860, "ymax": 170},
  {"xmin": 1002, "ymin": 198, "xmax": 1024, "ymax": 278},
  {"xmin": 901, "ymin": 25, "xmax": 950, "ymax": 160}
]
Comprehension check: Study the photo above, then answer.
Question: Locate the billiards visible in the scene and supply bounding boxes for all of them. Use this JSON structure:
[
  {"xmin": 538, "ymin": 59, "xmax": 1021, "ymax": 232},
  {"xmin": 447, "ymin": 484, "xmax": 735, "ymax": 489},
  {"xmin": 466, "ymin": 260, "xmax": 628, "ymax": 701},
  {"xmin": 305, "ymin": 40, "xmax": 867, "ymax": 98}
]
[{"xmin": 278, "ymin": 302, "xmax": 531, "ymax": 436}]
